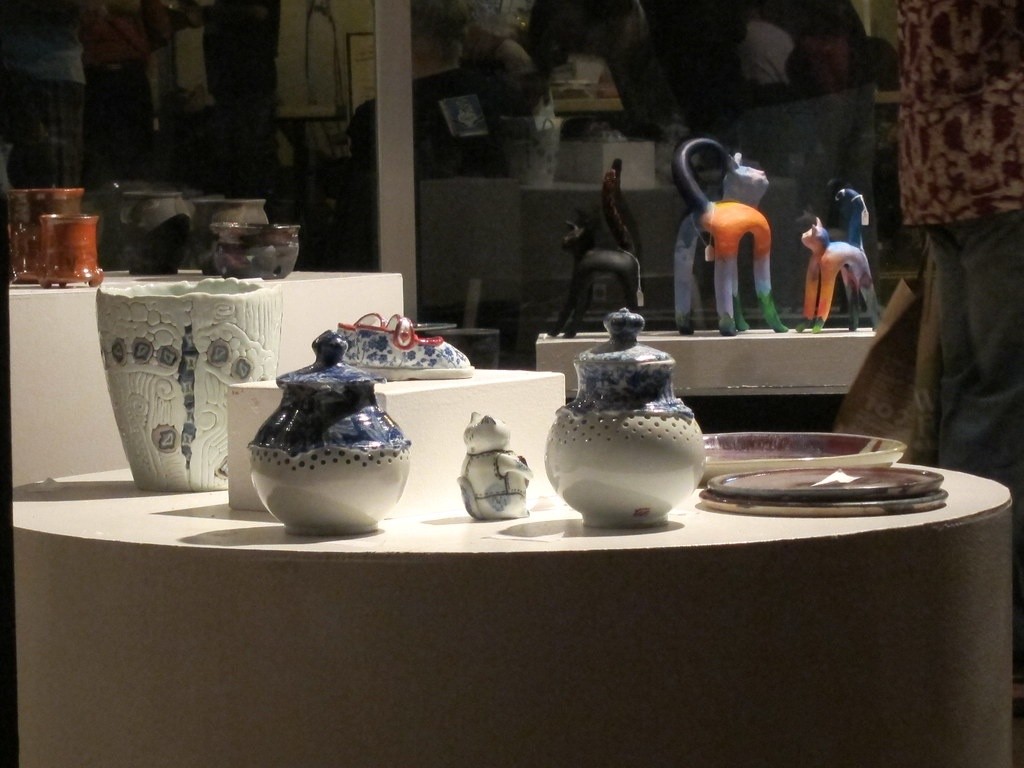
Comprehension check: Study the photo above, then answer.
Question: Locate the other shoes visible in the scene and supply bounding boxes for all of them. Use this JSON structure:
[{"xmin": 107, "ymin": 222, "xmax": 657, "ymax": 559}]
[{"xmin": 336, "ymin": 311, "xmax": 477, "ymax": 380}]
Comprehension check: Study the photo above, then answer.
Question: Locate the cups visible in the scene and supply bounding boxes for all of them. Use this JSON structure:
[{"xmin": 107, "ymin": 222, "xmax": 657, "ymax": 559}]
[{"xmin": 501, "ymin": 113, "xmax": 563, "ymax": 184}]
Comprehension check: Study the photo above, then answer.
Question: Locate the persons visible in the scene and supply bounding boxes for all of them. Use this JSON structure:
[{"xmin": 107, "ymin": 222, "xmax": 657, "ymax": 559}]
[
  {"xmin": 0, "ymin": 0, "xmax": 905, "ymax": 270},
  {"xmin": 894, "ymin": 1, "xmax": 1024, "ymax": 716}
]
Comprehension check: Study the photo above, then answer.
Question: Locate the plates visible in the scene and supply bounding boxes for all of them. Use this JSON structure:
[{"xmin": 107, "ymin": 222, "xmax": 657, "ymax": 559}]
[
  {"xmin": 699, "ymin": 490, "xmax": 949, "ymax": 518},
  {"xmin": 707, "ymin": 467, "xmax": 944, "ymax": 501},
  {"xmin": 698, "ymin": 433, "xmax": 908, "ymax": 485}
]
